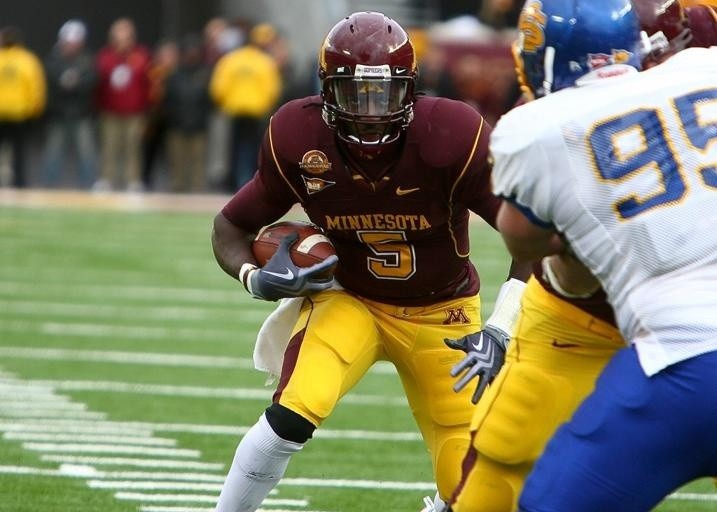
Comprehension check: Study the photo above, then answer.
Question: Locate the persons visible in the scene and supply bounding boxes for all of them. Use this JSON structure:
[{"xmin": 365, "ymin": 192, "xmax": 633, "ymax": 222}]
[
  {"xmin": 422, "ymin": 1, "xmax": 717, "ymax": 511},
  {"xmin": 1, "ymin": 15, "xmax": 284, "ymax": 195},
  {"xmin": 211, "ymin": 12, "xmax": 562, "ymax": 510}
]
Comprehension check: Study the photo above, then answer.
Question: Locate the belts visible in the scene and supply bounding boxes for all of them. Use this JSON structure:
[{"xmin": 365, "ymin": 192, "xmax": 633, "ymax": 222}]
[
  {"xmin": 362, "ymin": 298, "xmax": 442, "ymax": 318},
  {"xmin": 540, "ymin": 289, "xmax": 625, "ymax": 346}
]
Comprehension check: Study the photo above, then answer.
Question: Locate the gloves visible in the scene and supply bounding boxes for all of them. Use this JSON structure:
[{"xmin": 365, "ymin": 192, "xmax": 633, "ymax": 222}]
[
  {"xmin": 251, "ymin": 232, "xmax": 340, "ymax": 302},
  {"xmin": 444, "ymin": 325, "xmax": 510, "ymax": 406}
]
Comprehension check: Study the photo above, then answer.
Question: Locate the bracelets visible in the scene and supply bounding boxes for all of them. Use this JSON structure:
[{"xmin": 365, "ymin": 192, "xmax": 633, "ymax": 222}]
[
  {"xmin": 239, "ymin": 262, "xmax": 258, "ymax": 297},
  {"xmin": 484, "ymin": 278, "xmax": 529, "ymax": 337},
  {"xmin": 542, "ymin": 259, "xmax": 600, "ymax": 302}
]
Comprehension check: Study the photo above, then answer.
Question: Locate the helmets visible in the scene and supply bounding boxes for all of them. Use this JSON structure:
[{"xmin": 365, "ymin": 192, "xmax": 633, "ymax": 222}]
[
  {"xmin": 320, "ymin": 11, "xmax": 419, "ymax": 185},
  {"xmin": 511, "ymin": 0, "xmax": 694, "ymax": 99}
]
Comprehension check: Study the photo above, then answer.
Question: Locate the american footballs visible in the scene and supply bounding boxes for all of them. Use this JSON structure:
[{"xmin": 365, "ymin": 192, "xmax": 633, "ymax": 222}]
[{"xmin": 252, "ymin": 221, "xmax": 338, "ymax": 280}]
